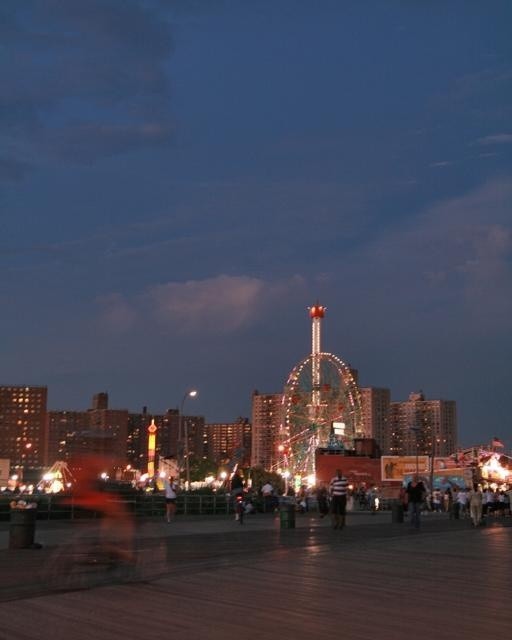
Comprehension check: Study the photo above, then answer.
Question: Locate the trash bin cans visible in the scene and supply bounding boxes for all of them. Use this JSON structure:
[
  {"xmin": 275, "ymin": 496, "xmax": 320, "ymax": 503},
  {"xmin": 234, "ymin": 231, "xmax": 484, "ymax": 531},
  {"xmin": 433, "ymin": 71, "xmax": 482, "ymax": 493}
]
[
  {"xmin": 10, "ymin": 508, "xmax": 42, "ymax": 549},
  {"xmin": 280, "ymin": 504, "xmax": 295, "ymax": 528},
  {"xmin": 391, "ymin": 504, "xmax": 403, "ymax": 522}
]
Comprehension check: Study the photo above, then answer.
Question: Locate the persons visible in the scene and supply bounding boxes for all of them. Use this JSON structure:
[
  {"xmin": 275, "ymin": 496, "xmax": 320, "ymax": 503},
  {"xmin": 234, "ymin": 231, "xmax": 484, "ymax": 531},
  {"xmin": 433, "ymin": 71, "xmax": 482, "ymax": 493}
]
[
  {"xmin": 165, "ymin": 475, "xmax": 177, "ymax": 523},
  {"xmin": 230, "ymin": 464, "xmax": 512, "ymax": 531}
]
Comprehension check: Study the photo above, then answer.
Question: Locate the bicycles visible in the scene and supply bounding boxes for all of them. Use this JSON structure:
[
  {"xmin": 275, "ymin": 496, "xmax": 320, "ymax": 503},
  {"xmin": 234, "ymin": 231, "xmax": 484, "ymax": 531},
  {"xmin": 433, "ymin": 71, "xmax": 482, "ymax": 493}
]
[{"xmin": 230, "ymin": 490, "xmax": 245, "ymax": 525}]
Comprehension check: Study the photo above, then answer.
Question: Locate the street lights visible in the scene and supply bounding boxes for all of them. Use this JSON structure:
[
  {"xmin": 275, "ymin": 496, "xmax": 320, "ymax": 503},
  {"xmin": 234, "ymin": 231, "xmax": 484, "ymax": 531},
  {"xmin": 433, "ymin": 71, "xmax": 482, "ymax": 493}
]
[{"xmin": 178, "ymin": 389, "xmax": 197, "ymax": 441}]
